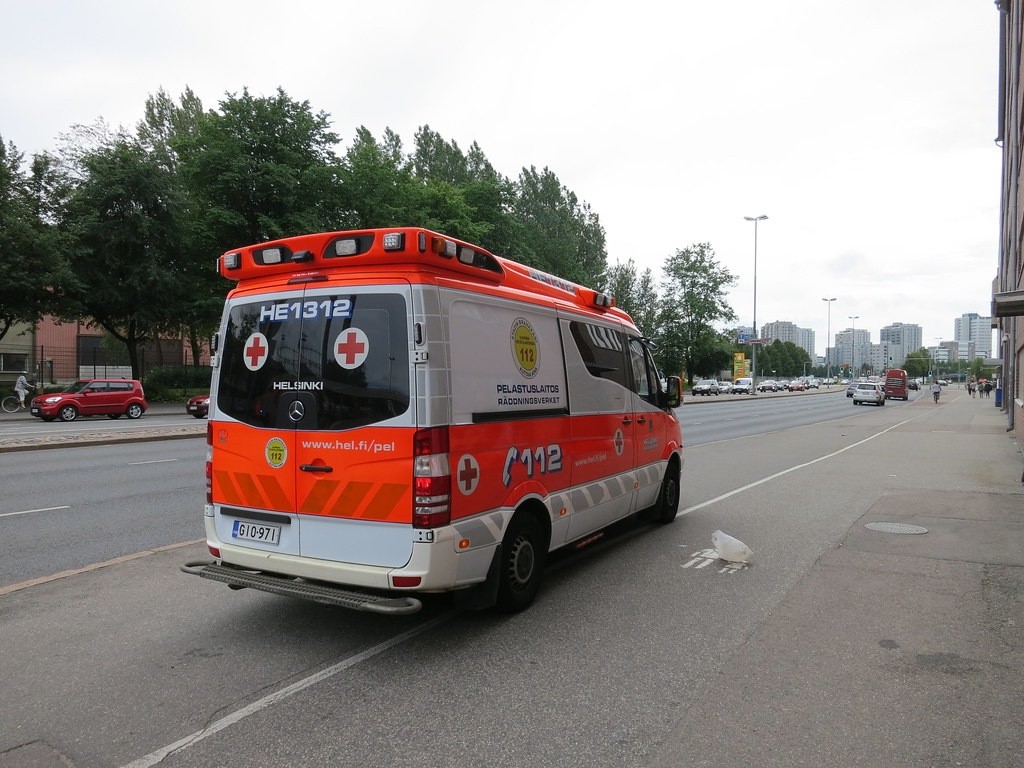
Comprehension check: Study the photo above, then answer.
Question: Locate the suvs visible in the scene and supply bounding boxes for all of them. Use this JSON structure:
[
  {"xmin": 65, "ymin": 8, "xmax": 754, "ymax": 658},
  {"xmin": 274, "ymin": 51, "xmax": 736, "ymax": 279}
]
[
  {"xmin": 691, "ymin": 378, "xmax": 720, "ymax": 396},
  {"xmin": 853, "ymin": 383, "xmax": 885, "ymax": 406}
]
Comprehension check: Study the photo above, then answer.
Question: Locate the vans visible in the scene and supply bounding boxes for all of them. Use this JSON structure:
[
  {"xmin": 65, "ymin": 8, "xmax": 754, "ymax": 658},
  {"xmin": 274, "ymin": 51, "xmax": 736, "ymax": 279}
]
[
  {"xmin": 733, "ymin": 378, "xmax": 757, "ymax": 394},
  {"xmin": 884, "ymin": 369, "xmax": 909, "ymax": 401}
]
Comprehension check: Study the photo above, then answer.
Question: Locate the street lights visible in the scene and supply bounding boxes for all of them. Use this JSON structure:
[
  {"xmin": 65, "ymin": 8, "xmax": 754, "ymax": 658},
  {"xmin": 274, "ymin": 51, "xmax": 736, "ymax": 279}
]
[
  {"xmin": 743, "ymin": 212, "xmax": 769, "ymax": 396},
  {"xmin": 822, "ymin": 296, "xmax": 837, "ymax": 389},
  {"xmin": 848, "ymin": 314, "xmax": 859, "ymax": 381},
  {"xmin": 934, "ymin": 336, "xmax": 943, "ymax": 380}
]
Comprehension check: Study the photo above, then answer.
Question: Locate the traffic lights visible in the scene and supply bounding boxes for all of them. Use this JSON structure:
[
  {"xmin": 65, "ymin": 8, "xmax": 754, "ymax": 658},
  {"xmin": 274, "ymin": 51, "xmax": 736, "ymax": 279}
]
[
  {"xmin": 928, "ymin": 371, "xmax": 932, "ymax": 378},
  {"xmin": 840, "ymin": 367, "xmax": 843, "ymax": 373},
  {"xmin": 849, "ymin": 369, "xmax": 851, "ymax": 374},
  {"xmin": 889, "ymin": 357, "xmax": 892, "ymax": 362}
]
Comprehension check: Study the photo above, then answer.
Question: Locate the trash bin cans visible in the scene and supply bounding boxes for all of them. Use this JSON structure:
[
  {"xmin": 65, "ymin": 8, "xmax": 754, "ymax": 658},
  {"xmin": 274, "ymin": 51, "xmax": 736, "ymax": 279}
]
[{"xmin": 995, "ymin": 388, "xmax": 1003, "ymax": 406}]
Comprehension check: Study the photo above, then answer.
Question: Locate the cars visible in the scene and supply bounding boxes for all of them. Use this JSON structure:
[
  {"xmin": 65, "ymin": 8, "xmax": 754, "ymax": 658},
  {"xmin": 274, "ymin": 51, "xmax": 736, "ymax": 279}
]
[
  {"xmin": 908, "ymin": 380, "xmax": 922, "ymax": 391},
  {"xmin": 781, "ymin": 380, "xmax": 790, "ymax": 389},
  {"xmin": 938, "ymin": 378, "xmax": 953, "ymax": 386},
  {"xmin": 759, "ymin": 379, "xmax": 777, "ymax": 392},
  {"xmin": 31, "ymin": 377, "xmax": 149, "ymax": 422},
  {"xmin": 186, "ymin": 391, "xmax": 211, "ymax": 418},
  {"xmin": 718, "ymin": 381, "xmax": 734, "ymax": 394},
  {"xmin": 788, "ymin": 380, "xmax": 805, "ymax": 393},
  {"xmin": 757, "ymin": 381, "xmax": 763, "ymax": 390},
  {"xmin": 777, "ymin": 381, "xmax": 784, "ymax": 391},
  {"xmin": 808, "ymin": 379, "xmax": 820, "ymax": 389},
  {"xmin": 847, "ymin": 382, "xmax": 861, "ymax": 397},
  {"xmin": 823, "ymin": 377, "xmax": 834, "ymax": 385}
]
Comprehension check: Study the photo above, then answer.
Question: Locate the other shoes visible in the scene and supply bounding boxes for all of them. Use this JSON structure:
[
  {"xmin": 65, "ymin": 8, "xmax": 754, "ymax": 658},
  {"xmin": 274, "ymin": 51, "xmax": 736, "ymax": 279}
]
[{"xmin": 21, "ymin": 404, "xmax": 26, "ymax": 408}]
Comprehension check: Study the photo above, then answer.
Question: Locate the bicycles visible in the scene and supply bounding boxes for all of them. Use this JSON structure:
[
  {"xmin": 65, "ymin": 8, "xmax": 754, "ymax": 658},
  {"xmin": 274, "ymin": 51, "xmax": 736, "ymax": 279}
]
[
  {"xmin": 934, "ymin": 392, "xmax": 939, "ymax": 404},
  {"xmin": 1, "ymin": 385, "xmax": 44, "ymax": 414}
]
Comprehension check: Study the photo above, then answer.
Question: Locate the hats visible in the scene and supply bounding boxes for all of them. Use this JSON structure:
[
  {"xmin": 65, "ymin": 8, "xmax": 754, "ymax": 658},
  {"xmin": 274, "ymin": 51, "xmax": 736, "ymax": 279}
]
[{"xmin": 21, "ymin": 370, "xmax": 28, "ymax": 374}]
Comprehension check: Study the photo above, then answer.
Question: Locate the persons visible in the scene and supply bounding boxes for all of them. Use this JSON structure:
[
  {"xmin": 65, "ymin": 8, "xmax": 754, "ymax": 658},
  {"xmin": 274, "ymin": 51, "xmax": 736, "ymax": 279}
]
[
  {"xmin": 932, "ymin": 381, "xmax": 941, "ymax": 401},
  {"xmin": 982, "ymin": 382, "xmax": 986, "ymax": 390},
  {"xmin": 985, "ymin": 382, "xmax": 990, "ymax": 397},
  {"xmin": 970, "ymin": 381, "xmax": 976, "ymax": 398},
  {"xmin": 978, "ymin": 382, "xmax": 984, "ymax": 398},
  {"xmin": 967, "ymin": 382, "xmax": 971, "ymax": 395},
  {"xmin": 14, "ymin": 371, "xmax": 34, "ymax": 409}
]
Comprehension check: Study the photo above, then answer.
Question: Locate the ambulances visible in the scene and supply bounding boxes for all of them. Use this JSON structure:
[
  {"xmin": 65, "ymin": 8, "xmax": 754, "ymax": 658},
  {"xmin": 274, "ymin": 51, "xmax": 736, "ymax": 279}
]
[{"xmin": 172, "ymin": 221, "xmax": 688, "ymax": 622}]
